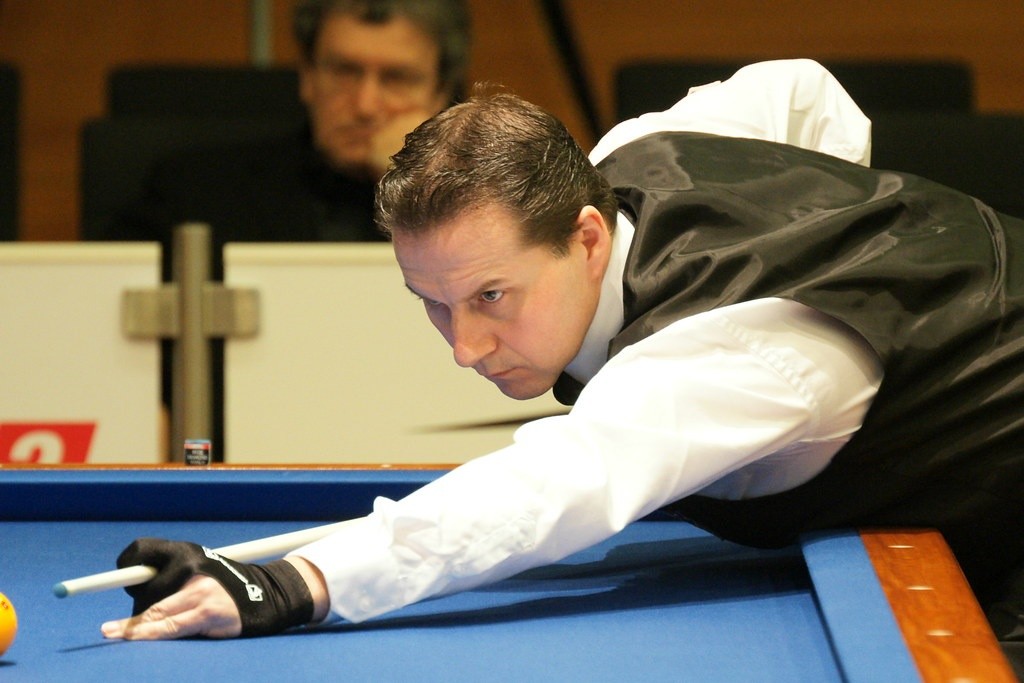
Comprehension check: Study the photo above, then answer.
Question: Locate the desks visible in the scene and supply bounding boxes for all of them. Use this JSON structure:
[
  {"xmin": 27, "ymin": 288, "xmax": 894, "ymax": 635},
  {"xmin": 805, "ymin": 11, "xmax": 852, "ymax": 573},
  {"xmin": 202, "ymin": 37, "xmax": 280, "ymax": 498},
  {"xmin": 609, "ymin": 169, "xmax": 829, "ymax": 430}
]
[
  {"xmin": 2, "ymin": 459, "xmax": 1020, "ymax": 683},
  {"xmin": 0, "ymin": 241, "xmax": 573, "ymax": 462}
]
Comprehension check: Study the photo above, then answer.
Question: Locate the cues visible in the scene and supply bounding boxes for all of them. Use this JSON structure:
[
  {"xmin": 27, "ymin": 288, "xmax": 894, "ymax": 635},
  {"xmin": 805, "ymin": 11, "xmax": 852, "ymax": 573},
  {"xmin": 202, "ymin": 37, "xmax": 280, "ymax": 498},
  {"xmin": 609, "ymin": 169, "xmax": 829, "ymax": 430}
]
[{"xmin": 51, "ymin": 510, "xmax": 378, "ymax": 604}]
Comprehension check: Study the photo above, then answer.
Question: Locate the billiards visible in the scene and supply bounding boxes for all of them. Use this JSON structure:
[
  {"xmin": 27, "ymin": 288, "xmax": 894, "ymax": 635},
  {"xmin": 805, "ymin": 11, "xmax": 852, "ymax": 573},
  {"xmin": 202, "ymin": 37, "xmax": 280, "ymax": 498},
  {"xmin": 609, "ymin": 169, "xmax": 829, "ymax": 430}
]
[{"xmin": 0, "ymin": 591, "xmax": 18, "ymax": 660}]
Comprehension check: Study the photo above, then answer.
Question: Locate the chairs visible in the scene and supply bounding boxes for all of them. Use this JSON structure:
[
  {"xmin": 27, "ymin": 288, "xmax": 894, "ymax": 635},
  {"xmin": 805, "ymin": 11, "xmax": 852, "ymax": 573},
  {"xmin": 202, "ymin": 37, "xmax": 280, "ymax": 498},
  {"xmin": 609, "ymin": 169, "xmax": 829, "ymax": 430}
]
[
  {"xmin": 616, "ymin": 54, "xmax": 977, "ymax": 119},
  {"xmin": 79, "ymin": 60, "xmax": 466, "ymax": 243}
]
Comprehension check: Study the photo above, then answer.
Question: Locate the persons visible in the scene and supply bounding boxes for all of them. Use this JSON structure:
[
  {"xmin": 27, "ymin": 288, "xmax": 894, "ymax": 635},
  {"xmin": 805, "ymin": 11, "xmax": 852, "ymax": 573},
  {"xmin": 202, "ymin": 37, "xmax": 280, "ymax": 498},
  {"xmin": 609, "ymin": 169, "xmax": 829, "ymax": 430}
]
[
  {"xmin": 109, "ymin": 0, "xmax": 472, "ymax": 244},
  {"xmin": 101, "ymin": 58, "xmax": 1023, "ymax": 683}
]
